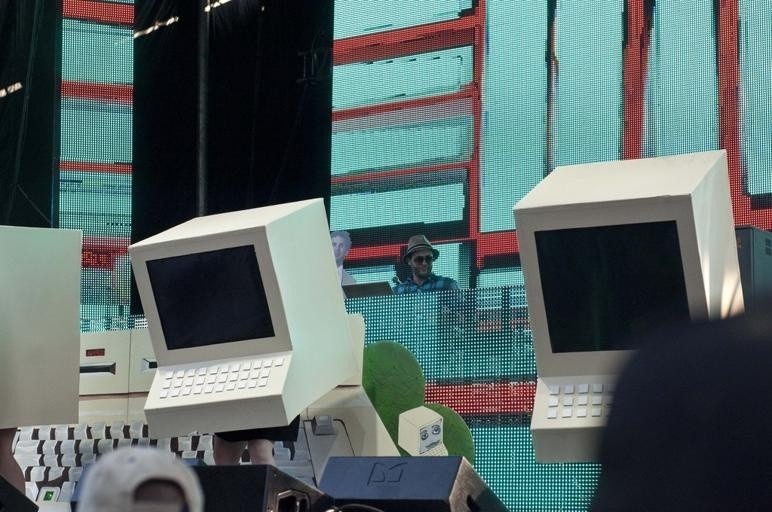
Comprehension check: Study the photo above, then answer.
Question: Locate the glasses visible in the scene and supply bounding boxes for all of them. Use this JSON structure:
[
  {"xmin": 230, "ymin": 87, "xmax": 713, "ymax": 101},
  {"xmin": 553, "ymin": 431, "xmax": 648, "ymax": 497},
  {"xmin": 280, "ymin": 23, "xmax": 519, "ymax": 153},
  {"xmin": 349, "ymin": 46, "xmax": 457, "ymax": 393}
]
[{"xmin": 413, "ymin": 255, "xmax": 434, "ymax": 264}]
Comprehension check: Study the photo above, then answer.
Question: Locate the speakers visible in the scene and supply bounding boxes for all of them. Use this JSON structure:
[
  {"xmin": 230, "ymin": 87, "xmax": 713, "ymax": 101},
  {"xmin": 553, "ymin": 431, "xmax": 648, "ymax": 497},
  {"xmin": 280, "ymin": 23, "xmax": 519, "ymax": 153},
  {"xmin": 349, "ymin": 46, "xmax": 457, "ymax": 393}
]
[
  {"xmin": 192, "ymin": 464, "xmax": 323, "ymax": 512},
  {"xmin": 319, "ymin": 452, "xmax": 512, "ymax": 512}
]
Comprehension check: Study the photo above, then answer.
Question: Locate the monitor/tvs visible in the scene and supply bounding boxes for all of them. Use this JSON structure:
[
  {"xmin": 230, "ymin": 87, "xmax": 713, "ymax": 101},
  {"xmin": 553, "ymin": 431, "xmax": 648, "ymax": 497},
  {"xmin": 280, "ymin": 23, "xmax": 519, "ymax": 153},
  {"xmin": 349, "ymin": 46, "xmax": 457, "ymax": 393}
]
[
  {"xmin": 507, "ymin": 146, "xmax": 745, "ymax": 378},
  {"xmin": 127, "ymin": 198, "xmax": 348, "ymax": 365}
]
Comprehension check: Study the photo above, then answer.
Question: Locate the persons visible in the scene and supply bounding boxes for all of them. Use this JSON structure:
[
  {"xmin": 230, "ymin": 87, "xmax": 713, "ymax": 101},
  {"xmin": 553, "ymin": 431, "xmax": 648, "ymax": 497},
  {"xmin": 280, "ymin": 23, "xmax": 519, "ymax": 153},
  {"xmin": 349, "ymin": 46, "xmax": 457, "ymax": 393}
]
[
  {"xmin": 330, "ymin": 230, "xmax": 357, "ymax": 286},
  {"xmin": 591, "ymin": 311, "xmax": 772, "ymax": 511},
  {"xmin": 393, "ymin": 234, "xmax": 479, "ymax": 332}
]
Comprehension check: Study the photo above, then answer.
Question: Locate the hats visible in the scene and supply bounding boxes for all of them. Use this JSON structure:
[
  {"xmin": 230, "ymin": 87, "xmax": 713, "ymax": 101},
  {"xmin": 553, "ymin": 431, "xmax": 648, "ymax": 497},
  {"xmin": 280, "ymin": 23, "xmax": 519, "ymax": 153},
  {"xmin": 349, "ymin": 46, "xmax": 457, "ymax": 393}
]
[{"xmin": 402, "ymin": 234, "xmax": 439, "ymax": 264}]
